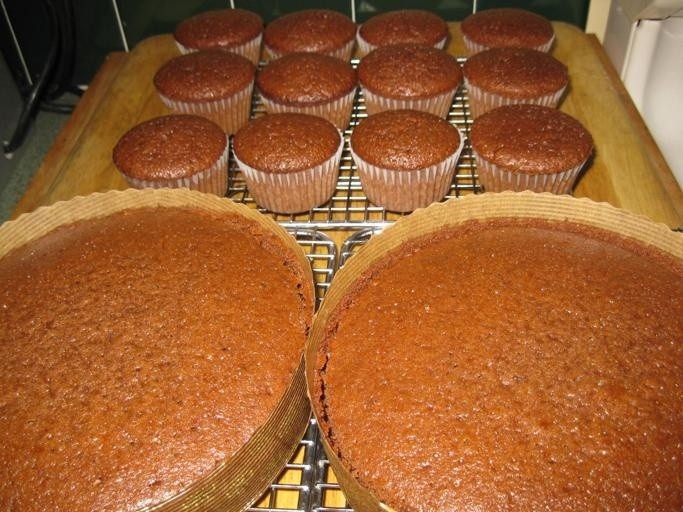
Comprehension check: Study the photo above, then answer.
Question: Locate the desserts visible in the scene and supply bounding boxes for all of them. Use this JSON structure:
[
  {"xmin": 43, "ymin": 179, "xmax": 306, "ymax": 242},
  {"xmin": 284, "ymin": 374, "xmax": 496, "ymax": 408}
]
[{"xmin": 110, "ymin": 8, "xmax": 595, "ymax": 215}]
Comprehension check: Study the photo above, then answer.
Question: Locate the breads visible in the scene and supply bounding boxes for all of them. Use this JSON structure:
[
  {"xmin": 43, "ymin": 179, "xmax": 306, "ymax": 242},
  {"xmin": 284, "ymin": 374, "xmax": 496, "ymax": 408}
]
[
  {"xmin": 311, "ymin": 216, "xmax": 683, "ymax": 512},
  {"xmin": 0, "ymin": 207, "xmax": 310, "ymax": 512}
]
